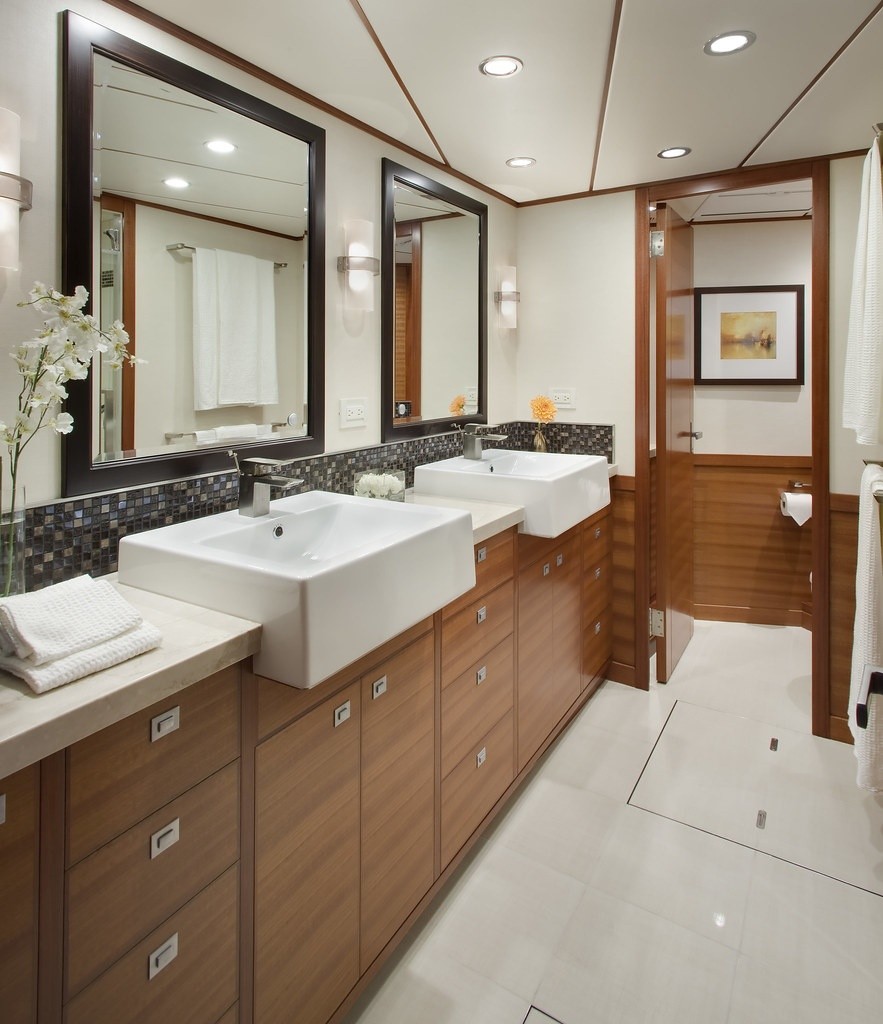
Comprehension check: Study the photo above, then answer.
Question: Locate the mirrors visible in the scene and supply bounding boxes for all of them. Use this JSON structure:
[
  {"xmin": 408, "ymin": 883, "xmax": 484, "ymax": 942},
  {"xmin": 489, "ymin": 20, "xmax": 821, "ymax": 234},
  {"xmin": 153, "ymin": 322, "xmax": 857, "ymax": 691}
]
[
  {"xmin": 380, "ymin": 156, "xmax": 488, "ymax": 437},
  {"xmin": 60, "ymin": 4, "xmax": 325, "ymax": 499}
]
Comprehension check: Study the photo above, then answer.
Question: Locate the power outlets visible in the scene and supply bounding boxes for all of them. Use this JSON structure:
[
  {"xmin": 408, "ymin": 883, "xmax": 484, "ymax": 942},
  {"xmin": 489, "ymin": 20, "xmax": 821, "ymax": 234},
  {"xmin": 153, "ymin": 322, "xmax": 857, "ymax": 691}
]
[{"xmin": 550, "ymin": 389, "xmax": 576, "ymax": 409}]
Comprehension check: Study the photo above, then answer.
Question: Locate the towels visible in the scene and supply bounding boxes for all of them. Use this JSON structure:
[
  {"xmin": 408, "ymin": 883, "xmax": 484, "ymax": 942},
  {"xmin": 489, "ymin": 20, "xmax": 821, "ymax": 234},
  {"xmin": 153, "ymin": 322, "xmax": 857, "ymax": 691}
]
[
  {"xmin": 845, "ymin": 464, "xmax": 881, "ymax": 805},
  {"xmin": 842, "ymin": 139, "xmax": 881, "ymax": 447},
  {"xmin": 0, "ymin": 571, "xmax": 164, "ymax": 697},
  {"xmin": 191, "ymin": 425, "xmax": 272, "ymax": 443},
  {"xmin": 193, "ymin": 246, "xmax": 280, "ymax": 411}
]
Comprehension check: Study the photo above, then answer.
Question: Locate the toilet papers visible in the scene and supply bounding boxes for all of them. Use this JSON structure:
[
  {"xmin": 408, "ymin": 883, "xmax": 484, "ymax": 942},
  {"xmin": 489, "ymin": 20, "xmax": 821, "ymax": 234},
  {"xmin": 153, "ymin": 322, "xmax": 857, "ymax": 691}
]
[{"xmin": 780, "ymin": 491, "xmax": 814, "ymax": 525}]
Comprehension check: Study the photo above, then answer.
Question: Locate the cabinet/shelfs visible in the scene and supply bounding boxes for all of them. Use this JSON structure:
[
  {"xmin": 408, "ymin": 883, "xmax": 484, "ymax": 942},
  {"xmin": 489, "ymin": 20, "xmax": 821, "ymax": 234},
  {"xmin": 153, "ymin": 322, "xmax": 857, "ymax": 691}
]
[{"xmin": 0, "ymin": 501, "xmax": 615, "ymax": 1024}]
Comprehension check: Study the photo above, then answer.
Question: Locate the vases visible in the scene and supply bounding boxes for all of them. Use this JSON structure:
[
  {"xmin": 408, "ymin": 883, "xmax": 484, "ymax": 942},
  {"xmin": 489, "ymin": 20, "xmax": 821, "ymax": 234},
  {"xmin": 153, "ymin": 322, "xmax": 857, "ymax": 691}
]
[
  {"xmin": 532, "ymin": 427, "xmax": 548, "ymax": 454},
  {"xmin": 0, "ymin": 423, "xmax": 43, "ymax": 591}
]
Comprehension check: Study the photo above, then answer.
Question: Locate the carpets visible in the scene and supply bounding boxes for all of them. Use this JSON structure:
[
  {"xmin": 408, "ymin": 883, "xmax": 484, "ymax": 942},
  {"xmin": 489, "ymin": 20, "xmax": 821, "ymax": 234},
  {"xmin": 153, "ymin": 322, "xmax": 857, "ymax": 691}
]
[{"xmin": 627, "ymin": 698, "xmax": 883, "ymax": 897}]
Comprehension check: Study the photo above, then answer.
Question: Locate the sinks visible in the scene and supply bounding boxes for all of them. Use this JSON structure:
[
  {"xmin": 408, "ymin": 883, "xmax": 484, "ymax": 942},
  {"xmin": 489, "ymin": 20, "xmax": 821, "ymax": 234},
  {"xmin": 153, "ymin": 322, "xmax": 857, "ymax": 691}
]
[
  {"xmin": 415, "ymin": 446, "xmax": 613, "ymax": 538},
  {"xmin": 121, "ymin": 488, "xmax": 478, "ymax": 690}
]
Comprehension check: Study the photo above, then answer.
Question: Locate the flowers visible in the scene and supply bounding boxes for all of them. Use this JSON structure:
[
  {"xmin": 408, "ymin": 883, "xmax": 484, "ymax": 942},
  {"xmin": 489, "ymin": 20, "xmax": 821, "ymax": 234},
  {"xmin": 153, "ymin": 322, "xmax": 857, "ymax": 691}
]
[
  {"xmin": 0, "ymin": 284, "xmax": 139, "ymax": 596},
  {"xmin": 531, "ymin": 396, "xmax": 556, "ymax": 430}
]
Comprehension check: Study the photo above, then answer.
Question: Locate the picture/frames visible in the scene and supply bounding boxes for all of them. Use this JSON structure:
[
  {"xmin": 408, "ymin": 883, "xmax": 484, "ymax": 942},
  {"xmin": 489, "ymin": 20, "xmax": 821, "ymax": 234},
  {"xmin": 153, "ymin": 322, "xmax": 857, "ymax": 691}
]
[{"xmin": 692, "ymin": 283, "xmax": 806, "ymax": 386}]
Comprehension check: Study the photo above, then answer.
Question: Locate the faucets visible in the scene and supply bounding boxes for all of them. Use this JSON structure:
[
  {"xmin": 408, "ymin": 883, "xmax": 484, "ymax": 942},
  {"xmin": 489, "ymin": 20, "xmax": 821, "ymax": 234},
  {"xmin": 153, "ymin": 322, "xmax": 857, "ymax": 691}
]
[
  {"xmin": 237, "ymin": 457, "xmax": 303, "ymax": 516},
  {"xmin": 461, "ymin": 423, "xmax": 508, "ymax": 459}
]
